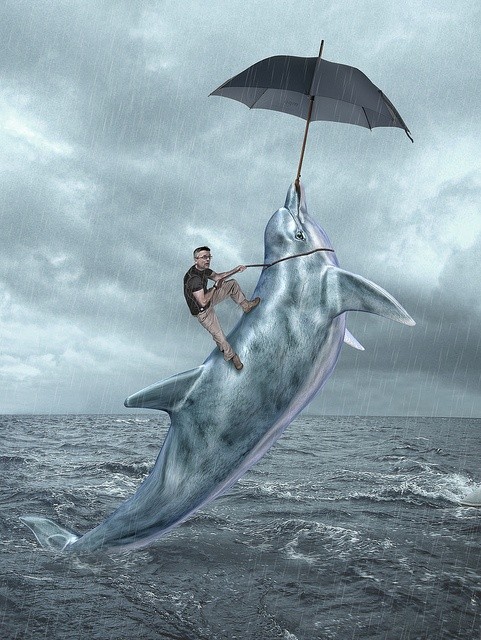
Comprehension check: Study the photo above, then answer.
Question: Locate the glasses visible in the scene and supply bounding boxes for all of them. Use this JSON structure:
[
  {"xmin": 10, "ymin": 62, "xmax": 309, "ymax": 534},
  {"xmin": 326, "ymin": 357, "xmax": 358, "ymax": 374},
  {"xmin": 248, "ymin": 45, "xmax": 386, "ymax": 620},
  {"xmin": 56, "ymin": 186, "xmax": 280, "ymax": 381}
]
[{"xmin": 194, "ymin": 255, "xmax": 212, "ymax": 259}]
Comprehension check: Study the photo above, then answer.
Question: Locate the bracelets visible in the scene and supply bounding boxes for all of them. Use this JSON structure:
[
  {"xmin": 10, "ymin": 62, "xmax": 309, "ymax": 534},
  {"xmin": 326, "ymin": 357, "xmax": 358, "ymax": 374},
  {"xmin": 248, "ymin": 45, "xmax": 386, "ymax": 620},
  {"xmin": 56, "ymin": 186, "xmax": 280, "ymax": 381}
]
[{"xmin": 213, "ymin": 285, "xmax": 217, "ymax": 290}]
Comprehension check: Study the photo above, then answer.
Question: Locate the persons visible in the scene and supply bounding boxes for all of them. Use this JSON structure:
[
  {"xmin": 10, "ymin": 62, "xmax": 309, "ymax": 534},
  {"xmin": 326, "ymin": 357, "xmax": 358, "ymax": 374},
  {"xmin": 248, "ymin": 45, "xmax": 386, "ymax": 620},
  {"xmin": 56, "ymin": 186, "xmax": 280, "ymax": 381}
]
[{"xmin": 183, "ymin": 246, "xmax": 260, "ymax": 370}]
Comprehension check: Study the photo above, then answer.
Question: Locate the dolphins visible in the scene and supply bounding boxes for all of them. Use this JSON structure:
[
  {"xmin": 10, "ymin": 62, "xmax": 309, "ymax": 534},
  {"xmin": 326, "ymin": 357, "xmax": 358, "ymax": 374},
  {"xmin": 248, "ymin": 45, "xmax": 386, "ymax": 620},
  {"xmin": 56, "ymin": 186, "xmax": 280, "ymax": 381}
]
[{"xmin": 14, "ymin": 180, "xmax": 418, "ymax": 572}]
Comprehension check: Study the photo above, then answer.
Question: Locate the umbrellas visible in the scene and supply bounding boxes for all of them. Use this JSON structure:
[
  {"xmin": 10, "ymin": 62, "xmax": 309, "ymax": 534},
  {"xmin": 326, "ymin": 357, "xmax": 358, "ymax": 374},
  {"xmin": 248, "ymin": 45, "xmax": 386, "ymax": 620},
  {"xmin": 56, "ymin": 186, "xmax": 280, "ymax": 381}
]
[{"xmin": 208, "ymin": 40, "xmax": 414, "ymax": 197}]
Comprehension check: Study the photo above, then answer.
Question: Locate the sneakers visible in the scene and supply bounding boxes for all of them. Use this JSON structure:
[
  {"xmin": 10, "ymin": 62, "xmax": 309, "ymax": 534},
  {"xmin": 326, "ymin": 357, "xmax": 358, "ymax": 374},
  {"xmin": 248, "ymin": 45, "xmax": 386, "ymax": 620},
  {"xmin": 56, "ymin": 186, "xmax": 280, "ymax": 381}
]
[
  {"xmin": 240, "ymin": 297, "xmax": 260, "ymax": 314},
  {"xmin": 232, "ymin": 354, "xmax": 243, "ymax": 370}
]
